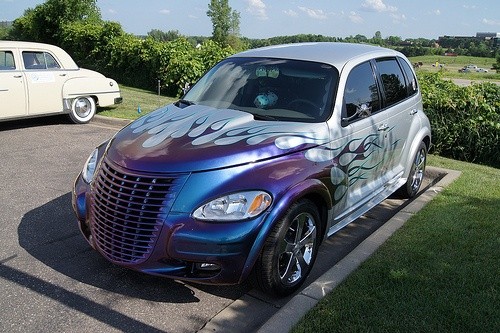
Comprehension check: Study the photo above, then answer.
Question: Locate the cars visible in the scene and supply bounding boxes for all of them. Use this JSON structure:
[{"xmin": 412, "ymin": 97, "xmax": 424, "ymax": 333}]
[
  {"xmin": 72, "ymin": 42, "xmax": 432, "ymax": 299},
  {"xmin": 459, "ymin": 68, "xmax": 471, "ymax": 73},
  {"xmin": 476, "ymin": 68, "xmax": 488, "ymax": 73},
  {"xmin": 0, "ymin": 41, "xmax": 123, "ymax": 124}
]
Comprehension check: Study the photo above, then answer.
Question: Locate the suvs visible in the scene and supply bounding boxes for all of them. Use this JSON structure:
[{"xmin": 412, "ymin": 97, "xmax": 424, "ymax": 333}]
[{"xmin": 465, "ymin": 64, "xmax": 477, "ymax": 69}]
